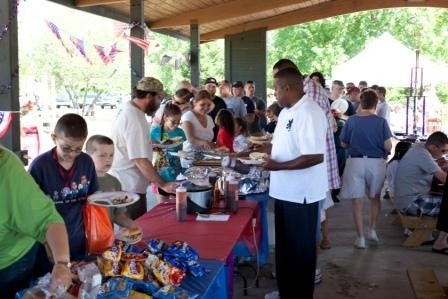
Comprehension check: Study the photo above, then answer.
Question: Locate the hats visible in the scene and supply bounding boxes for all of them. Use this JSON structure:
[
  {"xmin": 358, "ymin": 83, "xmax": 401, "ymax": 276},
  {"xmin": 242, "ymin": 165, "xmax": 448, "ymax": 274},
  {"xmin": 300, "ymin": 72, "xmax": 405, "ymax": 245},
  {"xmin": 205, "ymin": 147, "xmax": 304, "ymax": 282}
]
[
  {"xmin": 204, "ymin": 77, "xmax": 217, "ymax": 85},
  {"xmin": 137, "ymin": 76, "xmax": 172, "ymax": 98}
]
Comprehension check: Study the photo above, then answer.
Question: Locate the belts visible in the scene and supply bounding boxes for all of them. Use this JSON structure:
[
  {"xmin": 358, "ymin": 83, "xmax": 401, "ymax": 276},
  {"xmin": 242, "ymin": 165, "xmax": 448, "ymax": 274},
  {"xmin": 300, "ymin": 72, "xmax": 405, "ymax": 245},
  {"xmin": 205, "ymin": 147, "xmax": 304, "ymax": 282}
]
[{"xmin": 346, "ymin": 154, "xmax": 385, "ymax": 158}]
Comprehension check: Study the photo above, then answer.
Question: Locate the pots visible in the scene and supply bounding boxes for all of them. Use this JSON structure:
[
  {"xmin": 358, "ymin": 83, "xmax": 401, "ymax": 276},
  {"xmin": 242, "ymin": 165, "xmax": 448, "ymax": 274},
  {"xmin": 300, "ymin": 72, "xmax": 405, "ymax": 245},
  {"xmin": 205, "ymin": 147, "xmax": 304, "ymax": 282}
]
[{"xmin": 182, "ymin": 184, "xmax": 213, "ymax": 212}]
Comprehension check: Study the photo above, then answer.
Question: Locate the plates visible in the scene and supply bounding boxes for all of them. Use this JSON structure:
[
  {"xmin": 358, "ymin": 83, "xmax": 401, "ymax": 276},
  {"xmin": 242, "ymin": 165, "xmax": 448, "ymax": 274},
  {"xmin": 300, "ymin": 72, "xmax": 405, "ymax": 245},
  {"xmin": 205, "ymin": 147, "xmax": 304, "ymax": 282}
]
[
  {"xmin": 239, "ymin": 156, "xmax": 265, "ymax": 164},
  {"xmin": 154, "ymin": 141, "xmax": 182, "ymax": 147},
  {"xmin": 87, "ymin": 191, "xmax": 140, "ymax": 207},
  {"xmin": 330, "ymin": 99, "xmax": 349, "ymax": 113}
]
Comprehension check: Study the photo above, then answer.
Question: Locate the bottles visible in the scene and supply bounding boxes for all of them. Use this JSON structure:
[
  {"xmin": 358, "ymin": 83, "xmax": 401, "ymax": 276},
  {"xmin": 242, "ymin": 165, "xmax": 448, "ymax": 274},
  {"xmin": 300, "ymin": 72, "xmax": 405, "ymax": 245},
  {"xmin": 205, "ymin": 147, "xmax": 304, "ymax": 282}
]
[
  {"xmin": 175, "ymin": 184, "xmax": 187, "ymax": 221},
  {"xmin": 224, "ymin": 173, "xmax": 238, "ymax": 213}
]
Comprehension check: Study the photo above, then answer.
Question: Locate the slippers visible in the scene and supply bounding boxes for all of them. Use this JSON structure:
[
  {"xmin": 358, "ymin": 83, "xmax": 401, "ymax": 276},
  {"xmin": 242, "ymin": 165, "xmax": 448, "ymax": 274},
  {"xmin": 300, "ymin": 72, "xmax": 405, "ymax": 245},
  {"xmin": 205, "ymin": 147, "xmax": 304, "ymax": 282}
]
[{"xmin": 431, "ymin": 245, "xmax": 448, "ymax": 254}]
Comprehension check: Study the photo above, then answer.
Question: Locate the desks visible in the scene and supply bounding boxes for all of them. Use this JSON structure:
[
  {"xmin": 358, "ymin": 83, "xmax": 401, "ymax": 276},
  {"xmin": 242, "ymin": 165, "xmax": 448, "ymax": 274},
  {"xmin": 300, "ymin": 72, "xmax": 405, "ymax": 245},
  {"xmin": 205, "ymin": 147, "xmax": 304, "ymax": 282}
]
[{"xmin": 13, "ymin": 152, "xmax": 269, "ymax": 299}]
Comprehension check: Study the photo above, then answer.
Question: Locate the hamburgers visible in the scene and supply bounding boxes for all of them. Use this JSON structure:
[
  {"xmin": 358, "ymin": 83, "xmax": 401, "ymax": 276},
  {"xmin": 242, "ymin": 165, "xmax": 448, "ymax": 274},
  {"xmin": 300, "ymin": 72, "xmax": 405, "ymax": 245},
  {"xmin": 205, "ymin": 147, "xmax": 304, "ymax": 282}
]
[{"xmin": 120, "ymin": 225, "xmax": 142, "ymax": 244}]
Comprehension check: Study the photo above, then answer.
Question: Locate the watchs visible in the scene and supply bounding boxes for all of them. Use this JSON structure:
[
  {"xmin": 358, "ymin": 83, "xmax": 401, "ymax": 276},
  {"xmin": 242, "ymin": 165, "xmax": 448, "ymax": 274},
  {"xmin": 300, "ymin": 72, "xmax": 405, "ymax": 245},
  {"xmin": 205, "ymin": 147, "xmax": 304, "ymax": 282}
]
[{"xmin": 55, "ymin": 261, "xmax": 72, "ymax": 268}]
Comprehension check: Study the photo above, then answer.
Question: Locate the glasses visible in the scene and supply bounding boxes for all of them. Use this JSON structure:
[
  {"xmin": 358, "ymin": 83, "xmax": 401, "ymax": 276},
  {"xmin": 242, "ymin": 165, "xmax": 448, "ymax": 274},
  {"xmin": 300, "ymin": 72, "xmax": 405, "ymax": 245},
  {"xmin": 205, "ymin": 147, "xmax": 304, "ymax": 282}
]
[
  {"xmin": 168, "ymin": 117, "xmax": 183, "ymax": 125},
  {"xmin": 172, "ymin": 95, "xmax": 186, "ymax": 105},
  {"xmin": 56, "ymin": 137, "xmax": 83, "ymax": 153}
]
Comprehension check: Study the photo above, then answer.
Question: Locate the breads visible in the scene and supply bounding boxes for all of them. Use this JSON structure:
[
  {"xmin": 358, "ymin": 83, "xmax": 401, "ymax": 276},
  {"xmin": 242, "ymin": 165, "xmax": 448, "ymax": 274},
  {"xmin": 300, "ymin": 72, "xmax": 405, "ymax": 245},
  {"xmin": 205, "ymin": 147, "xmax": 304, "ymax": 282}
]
[
  {"xmin": 249, "ymin": 135, "xmax": 267, "ymax": 141},
  {"xmin": 249, "ymin": 152, "xmax": 267, "ymax": 160},
  {"xmin": 102, "ymin": 250, "xmax": 178, "ymax": 285}
]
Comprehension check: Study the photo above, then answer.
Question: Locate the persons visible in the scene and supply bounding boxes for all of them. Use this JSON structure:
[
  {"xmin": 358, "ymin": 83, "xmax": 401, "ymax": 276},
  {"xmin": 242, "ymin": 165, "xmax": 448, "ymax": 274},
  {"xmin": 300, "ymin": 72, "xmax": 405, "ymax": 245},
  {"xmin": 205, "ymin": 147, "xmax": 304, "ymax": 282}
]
[
  {"xmin": 250, "ymin": 102, "xmax": 280, "ymax": 141},
  {"xmin": 28, "ymin": 113, "xmax": 100, "ymax": 264},
  {"xmin": 0, "ymin": 144, "xmax": 72, "ymax": 299},
  {"xmin": 386, "ymin": 131, "xmax": 448, "ymax": 256},
  {"xmin": 310, "ymin": 71, "xmax": 392, "ymax": 250},
  {"xmin": 150, "ymin": 77, "xmax": 266, "ymax": 204},
  {"xmin": 266, "ymin": 65, "xmax": 328, "ymax": 299},
  {"xmin": 104, "ymin": 76, "xmax": 180, "ymax": 220},
  {"xmin": 86, "ymin": 135, "xmax": 135, "ymax": 232},
  {"xmin": 272, "ymin": 61, "xmax": 340, "ymax": 284}
]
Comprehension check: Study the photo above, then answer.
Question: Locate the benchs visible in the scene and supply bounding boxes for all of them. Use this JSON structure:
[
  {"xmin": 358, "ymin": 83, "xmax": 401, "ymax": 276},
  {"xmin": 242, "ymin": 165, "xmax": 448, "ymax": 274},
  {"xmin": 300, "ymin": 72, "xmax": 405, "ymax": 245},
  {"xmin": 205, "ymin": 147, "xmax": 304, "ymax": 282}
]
[{"xmin": 391, "ymin": 212, "xmax": 437, "ymax": 247}]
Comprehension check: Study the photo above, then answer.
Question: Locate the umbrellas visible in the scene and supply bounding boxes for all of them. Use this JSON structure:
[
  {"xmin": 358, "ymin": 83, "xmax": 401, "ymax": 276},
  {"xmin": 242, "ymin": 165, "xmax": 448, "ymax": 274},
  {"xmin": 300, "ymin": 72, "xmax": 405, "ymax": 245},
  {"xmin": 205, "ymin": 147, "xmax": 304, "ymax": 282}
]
[{"xmin": 331, "ymin": 32, "xmax": 448, "ymax": 87}]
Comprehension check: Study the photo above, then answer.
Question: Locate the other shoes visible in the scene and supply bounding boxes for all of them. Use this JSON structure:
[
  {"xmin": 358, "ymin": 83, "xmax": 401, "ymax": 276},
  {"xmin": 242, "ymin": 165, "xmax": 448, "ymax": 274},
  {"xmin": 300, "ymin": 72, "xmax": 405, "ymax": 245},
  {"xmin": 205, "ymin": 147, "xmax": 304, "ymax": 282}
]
[
  {"xmin": 367, "ymin": 228, "xmax": 378, "ymax": 241},
  {"xmin": 331, "ymin": 196, "xmax": 340, "ymax": 203},
  {"xmin": 264, "ymin": 291, "xmax": 280, "ymax": 299},
  {"xmin": 314, "ymin": 268, "xmax": 323, "ymax": 284},
  {"xmin": 354, "ymin": 236, "xmax": 366, "ymax": 249},
  {"xmin": 320, "ymin": 238, "xmax": 332, "ymax": 249}
]
